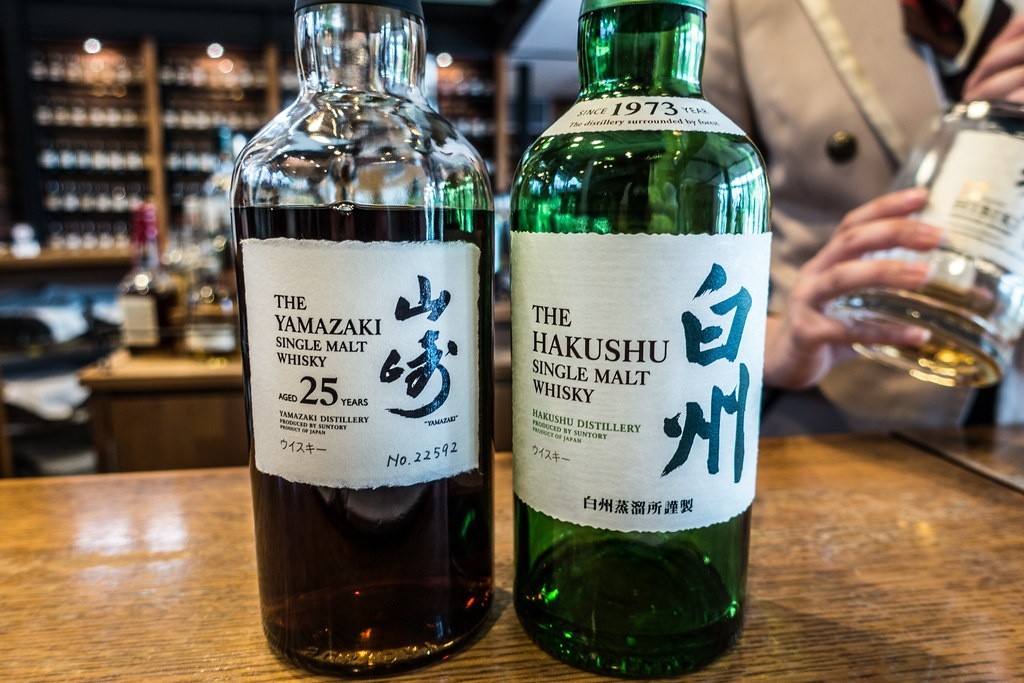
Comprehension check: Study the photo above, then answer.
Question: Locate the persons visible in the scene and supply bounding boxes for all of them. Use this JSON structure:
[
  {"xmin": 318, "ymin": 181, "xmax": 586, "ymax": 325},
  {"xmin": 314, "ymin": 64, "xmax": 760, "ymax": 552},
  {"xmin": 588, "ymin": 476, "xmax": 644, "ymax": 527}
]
[{"xmin": 696, "ymin": 0, "xmax": 1024, "ymax": 439}]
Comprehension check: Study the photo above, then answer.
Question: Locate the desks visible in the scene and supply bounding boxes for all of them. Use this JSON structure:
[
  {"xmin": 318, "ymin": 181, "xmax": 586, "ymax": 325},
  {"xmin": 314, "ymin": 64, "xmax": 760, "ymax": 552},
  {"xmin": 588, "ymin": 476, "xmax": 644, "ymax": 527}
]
[
  {"xmin": 0, "ymin": 422, "xmax": 1024, "ymax": 683},
  {"xmin": 79, "ymin": 301, "xmax": 513, "ymax": 473}
]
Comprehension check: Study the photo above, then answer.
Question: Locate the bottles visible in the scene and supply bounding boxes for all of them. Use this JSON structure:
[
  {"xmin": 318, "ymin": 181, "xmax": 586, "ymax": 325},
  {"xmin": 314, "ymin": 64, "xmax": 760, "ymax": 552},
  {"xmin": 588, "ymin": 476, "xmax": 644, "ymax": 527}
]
[
  {"xmin": 230, "ymin": 1, "xmax": 498, "ymax": 675},
  {"xmin": 836, "ymin": 98, "xmax": 1024, "ymax": 385},
  {"xmin": 30, "ymin": 48, "xmax": 267, "ymax": 250},
  {"xmin": 186, "ymin": 123, "xmax": 243, "ymax": 357},
  {"xmin": 437, "ymin": 69, "xmax": 495, "ymax": 189},
  {"xmin": 510, "ymin": 0, "xmax": 771, "ymax": 674},
  {"xmin": 119, "ymin": 201, "xmax": 186, "ymax": 354}
]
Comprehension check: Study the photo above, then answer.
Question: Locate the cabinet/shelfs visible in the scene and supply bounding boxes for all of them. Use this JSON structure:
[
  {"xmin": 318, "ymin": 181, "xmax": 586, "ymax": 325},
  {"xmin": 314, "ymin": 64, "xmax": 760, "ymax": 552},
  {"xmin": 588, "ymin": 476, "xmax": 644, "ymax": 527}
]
[{"xmin": 30, "ymin": 40, "xmax": 507, "ymax": 247}]
